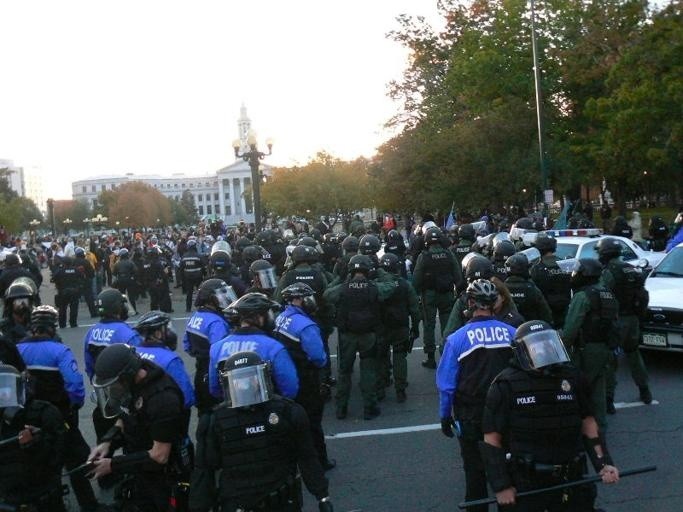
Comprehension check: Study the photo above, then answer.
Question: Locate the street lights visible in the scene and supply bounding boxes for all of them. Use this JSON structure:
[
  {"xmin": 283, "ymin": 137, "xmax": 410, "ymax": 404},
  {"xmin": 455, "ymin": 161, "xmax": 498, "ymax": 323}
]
[
  {"xmin": 62, "ymin": 218, "xmax": 72, "ymax": 238},
  {"xmin": 28, "ymin": 219, "xmax": 40, "ymax": 241},
  {"xmin": 115, "ymin": 221, "xmax": 120, "ymax": 234},
  {"xmin": 92, "ymin": 214, "xmax": 109, "ymax": 232},
  {"xmin": 125, "ymin": 217, "xmax": 129, "ymax": 234},
  {"xmin": 156, "ymin": 218, "xmax": 159, "ymax": 231},
  {"xmin": 81, "ymin": 218, "xmax": 93, "ymax": 239},
  {"xmin": 233, "ymin": 134, "xmax": 275, "ymax": 235}
]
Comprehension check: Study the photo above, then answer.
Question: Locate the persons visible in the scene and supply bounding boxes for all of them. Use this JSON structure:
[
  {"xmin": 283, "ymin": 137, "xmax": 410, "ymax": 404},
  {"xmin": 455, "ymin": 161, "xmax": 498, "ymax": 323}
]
[
  {"xmin": 0, "ymin": 196, "xmax": 337, "ymax": 512},
  {"xmin": 432, "ymin": 279, "xmax": 618, "ymax": 512},
  {"xmin": 318, "ymin": 208, "xmax": 683, "ymax": 419}
]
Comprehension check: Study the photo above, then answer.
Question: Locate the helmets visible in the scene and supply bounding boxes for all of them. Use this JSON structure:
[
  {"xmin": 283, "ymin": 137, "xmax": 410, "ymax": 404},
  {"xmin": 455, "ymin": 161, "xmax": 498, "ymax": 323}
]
[
  {"xmin": 94, "ymin": 343, "xmax": 140, "ymax": 391},
  {"xmin": 210, "ymin": 222, "xmax": 622, "ymax": 301},
  {"xmin": 6, "ymin": 240, "xmax": 197, "ymax": 265},
  {"xmin": 223, "ymin": 293, "xmax": 282, "ymax": 316},
  {"xmin": 218, "ymin": 351, "xmax": 273, "ymax": 409},
  {"xmin": 513, "ymin": 320, "xmax": 572, "ymax": 371},
  {"xmin": 5, "ymin": 284, "xmax": 34, "ymax": 301},
  {"xmin": 30, "ymin": 304, "xmax": 59, "ymax": 333},
  {"xmin": 96, "ymin": 289, "xmax": 130, "ymax": 322},
  {"xmin": 281, "ymin": 282, "xmax": 317, "ymax": 298},
  {"xmin": 614, "ymin": 215, "xmax": 665, "ymax": 224},
  {"xmin": 132, "ymin": 310, "xmax": 171, "ymax": 331},
  {"xmin": 195, "ymin": 278, "xmax": 236, "ymax": 306}
]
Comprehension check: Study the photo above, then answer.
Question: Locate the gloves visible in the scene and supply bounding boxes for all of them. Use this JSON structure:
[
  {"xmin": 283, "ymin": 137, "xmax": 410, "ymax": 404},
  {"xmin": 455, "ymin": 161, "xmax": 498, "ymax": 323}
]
[
  {"xmin": 318, "ymin": 502, "xmax": 334, "ymax": 512},
  {"xmin": 441, "ymin": 417, "xmax": 458, "ymax": 438}
]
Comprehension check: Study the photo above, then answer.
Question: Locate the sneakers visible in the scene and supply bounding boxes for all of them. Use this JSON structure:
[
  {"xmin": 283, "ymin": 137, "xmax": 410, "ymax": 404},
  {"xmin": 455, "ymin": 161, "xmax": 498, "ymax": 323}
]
[
  {"xmin": 326, "ymin": 353, "xmax": 438, "ymax": 421},
  {"xmin": 638, "ymin": 387, "xmax": 653, "ymax": 405},
  {"xmin": 605, "ymin": 403, "xmax": 616, "ymax": 417},
  {"xmin": 322, "ymin": 459, "xmax": 337, "ymax": 471}
]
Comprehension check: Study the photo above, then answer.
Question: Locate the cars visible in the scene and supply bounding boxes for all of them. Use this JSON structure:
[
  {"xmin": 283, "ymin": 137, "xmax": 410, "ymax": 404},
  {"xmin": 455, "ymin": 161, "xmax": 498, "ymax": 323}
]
[
  {"xmin": 638, "ymin": 241, "xmax": 682, "ymax": 356},
  {"xmin": 506, "ymin": 228, "xmax": 665, "ymax": 282}
]
